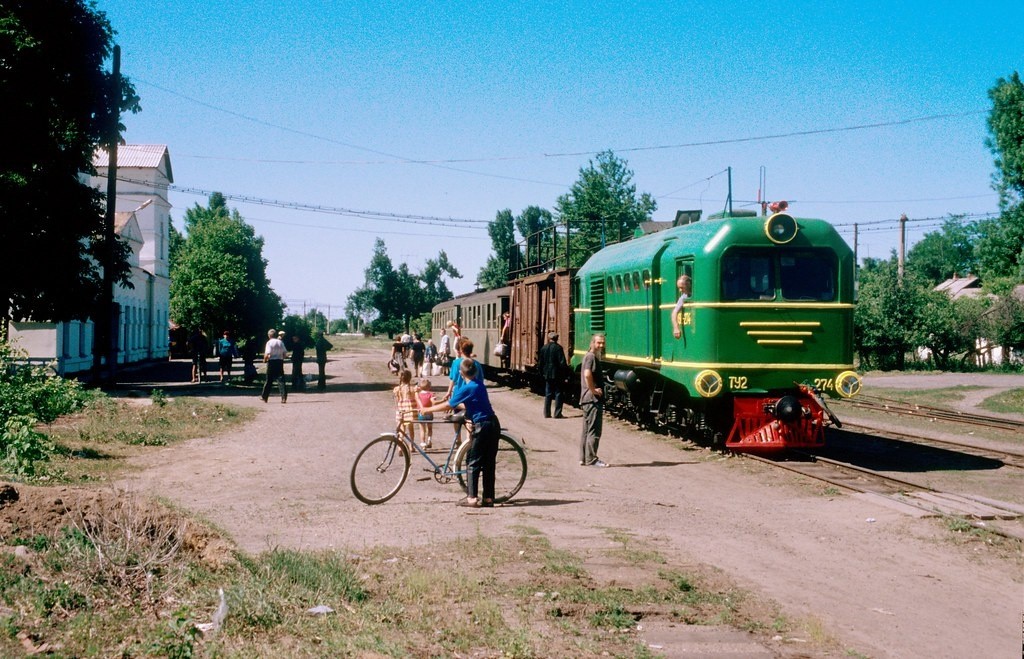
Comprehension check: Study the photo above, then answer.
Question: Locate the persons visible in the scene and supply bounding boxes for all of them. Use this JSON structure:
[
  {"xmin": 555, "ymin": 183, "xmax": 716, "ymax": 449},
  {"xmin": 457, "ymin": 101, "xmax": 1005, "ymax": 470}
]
[
  {"xmin": 536, "ymin": 332, "xmax": 569, "ymax": 419},
  {"xmin": 391, "ymin": 320, "xmax": 461, "ymax": 378},
  {"xmin": 392, "ymin": 369, "xmax": 446, "ymax": 456},
  {"xmin": 218, "ymin": 331, "xmax": 236, "ymax": 383},
  {"xmin": 578, "ymin": 334, "xmax": 610, "ymax": 467},
  {"xmin": 185, "ymin": 330, "xmax": 209, "ymax": 382},
  {"xmin": 500, "ymin": 311, "xmax": 510, "ymax": 342},
  {"xmin": 290, "ymin": 334, "xmax": 305, "ymax": 390},
  {"xmin": 315, "ymin": 329, "xmax": 333, "ymax": 389},
  {"xmin": 259, "ymin": 329, "xmax": 288, "ymax": 403},
  {"xmin": 419, "ymin": 358, "xmax": 501, "ymax": 507},
  {"xmin": 443, "ymin": 340, "xmax": 483, "ymax": 447},
  {"xmin": 671, "ymin": 275, "xmax": 692, "ymax": 338}
]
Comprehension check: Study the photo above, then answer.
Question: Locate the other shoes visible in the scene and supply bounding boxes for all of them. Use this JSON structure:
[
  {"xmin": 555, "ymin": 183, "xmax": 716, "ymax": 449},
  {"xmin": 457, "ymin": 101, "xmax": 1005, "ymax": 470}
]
[
  {"xmin": 593, "ymin": 460, "xmax": 609, "ymax": 468},
  {"xmin": 450, "ymin": 440, "xmax": 461, "ymax": 449},
  {"xmin": 281, "ymin": 399, "xmax": 286, "ymax": 403},
  {"xmin": 580, "ymin": 461, "xmax": 585, "ymax": 465},
  {"xmin": 560, "ymin": 415, "xmax": 569, "ymax": 419},
  {"xmin": 259, "ymin": 396, "xmax": 267, "ymax": 403},
  {"xmin": 426, "ymin": 440, "xmax": 432, "ymax": 448},
  {"xmin": 411, "ymin": 448, "xmax": 416, "ymax": 453},
  {"xmin": 421, "ymin": 446, "xmax": 426, "ymax": 450},
  {"xmin": 399, "ymin": 450, "xmax": 403, "ymax": 457}
]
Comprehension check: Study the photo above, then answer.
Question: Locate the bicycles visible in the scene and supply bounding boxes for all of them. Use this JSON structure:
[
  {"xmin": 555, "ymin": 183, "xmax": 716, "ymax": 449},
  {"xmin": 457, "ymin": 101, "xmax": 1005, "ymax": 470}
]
[{"xmin": 350, "ymin": 400, "xmax": 528, "ymax": 506}]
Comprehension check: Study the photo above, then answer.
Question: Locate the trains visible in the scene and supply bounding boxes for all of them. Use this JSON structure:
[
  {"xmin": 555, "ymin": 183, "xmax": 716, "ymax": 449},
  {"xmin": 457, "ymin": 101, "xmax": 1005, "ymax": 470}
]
[{"xmin": 431, "ymin": 165, "xmax": 862, "ymax": 455}]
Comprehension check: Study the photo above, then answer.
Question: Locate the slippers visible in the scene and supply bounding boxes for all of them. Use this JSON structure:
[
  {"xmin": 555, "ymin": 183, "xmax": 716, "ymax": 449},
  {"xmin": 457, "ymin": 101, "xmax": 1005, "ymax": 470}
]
[
  {"xmin": 478, "ymin": 499, "xmax": 494, "ymax": 508},
  {"xmin": 454, "ymin": 497, "xmax": 478, "ymax": 507}
]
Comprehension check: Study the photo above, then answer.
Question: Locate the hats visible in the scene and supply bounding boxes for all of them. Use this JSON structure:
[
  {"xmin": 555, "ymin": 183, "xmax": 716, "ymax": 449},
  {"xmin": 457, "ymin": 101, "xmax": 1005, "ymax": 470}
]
[{"xmin": 548, "ymin": 332, "xmax": 559, "ymax": 338}]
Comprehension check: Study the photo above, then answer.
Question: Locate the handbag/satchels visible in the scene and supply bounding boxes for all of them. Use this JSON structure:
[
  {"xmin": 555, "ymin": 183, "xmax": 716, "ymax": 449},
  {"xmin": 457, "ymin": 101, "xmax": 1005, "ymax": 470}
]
[
  {"xmin": 435, "ymin": 354, "xmax": 452, "ymax": 367},
  {"xmin": 388, "ymin": 359, "xmax": 400, "ymax": 374},
  {"xmin": 494, "ymin": 341, "xmax": 507, "ymax": 356}
]
